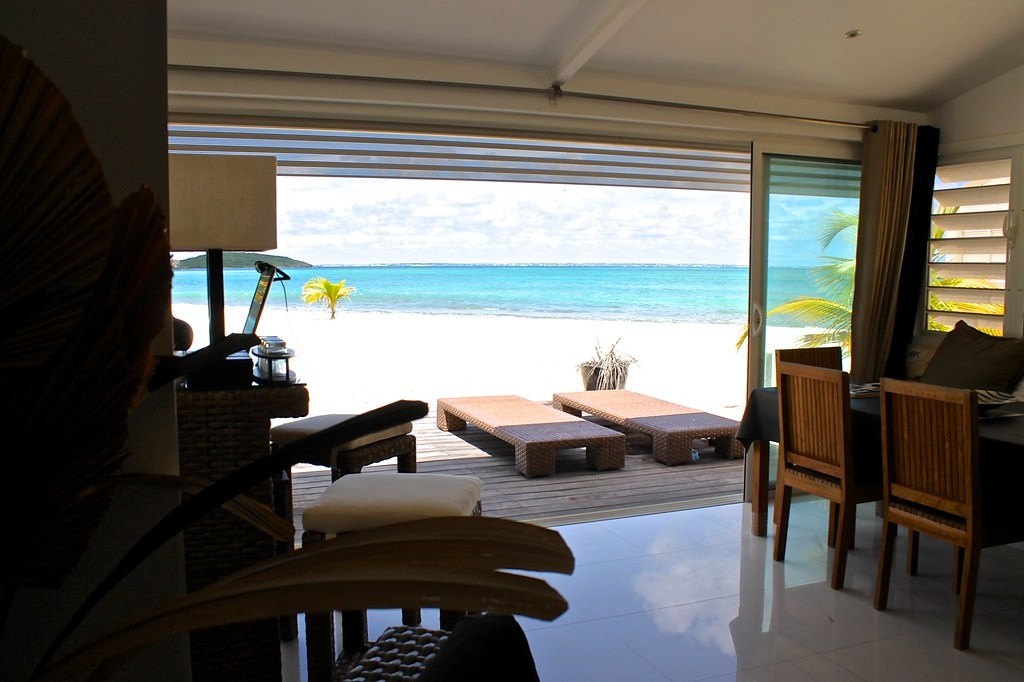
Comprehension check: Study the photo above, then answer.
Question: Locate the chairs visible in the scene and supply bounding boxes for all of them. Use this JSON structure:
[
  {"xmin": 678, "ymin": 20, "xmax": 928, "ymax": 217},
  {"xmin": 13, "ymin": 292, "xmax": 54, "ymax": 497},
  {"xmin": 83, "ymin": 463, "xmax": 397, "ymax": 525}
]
[
  {"xmin": 773, "ymin": 362, "xmax": 920, "ymax": 591},
  {"xmin": 773, "ymin": 346, "xmax": 858, "ymax": 551},
  {"xmin": 873, "ymin": 378, "xmax": 1024, "ymax": 651}
]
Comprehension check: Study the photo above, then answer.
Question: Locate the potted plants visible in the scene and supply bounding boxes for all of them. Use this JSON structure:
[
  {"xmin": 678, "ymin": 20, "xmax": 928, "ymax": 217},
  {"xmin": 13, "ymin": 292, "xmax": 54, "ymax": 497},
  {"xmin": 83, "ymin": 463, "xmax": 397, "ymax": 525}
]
[{"xmin": 576, "ymin": 335, "xmax": 641, "ymax": 391}]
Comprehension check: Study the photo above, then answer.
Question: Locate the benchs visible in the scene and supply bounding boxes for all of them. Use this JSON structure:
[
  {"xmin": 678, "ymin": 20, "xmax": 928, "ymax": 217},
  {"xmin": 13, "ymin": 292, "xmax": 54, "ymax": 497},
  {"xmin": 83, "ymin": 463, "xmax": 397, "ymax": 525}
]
[
  {"xmin": 436, "ymin": 394, "xmax": 627, "ymax": 479},
  {"xmin": 553, "ymin": 389, "xmax": 744, "ymax": 467}
]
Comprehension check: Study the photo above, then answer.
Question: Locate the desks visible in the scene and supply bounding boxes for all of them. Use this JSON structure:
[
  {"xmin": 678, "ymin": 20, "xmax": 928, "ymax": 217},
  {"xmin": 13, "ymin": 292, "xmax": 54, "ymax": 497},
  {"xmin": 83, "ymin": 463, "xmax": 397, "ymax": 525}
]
[
  {"xmin": 735, "ymin": 381, "xmax": 1024, "ymax": 537},
  {"xmin": 176, "ymin": 379, "xmax": 310, "ymax": 682}
]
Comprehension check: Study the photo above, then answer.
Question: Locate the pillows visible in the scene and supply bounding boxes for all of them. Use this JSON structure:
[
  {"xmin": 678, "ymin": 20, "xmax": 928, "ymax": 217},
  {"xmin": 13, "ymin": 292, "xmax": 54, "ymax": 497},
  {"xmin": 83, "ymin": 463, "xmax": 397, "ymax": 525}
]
[{"xmin": 916, "ymin": 320, "xmax": 1024, "ymax": 394}]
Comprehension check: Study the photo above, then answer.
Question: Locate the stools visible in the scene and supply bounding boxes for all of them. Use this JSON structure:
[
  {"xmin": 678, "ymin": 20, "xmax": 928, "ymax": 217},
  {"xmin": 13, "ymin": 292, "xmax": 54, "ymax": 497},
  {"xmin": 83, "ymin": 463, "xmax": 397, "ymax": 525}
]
[
  {"xmin": 301, "ymin": 473, "xmax": 473, "ymax": 682},
  {"xmin": 269, "ymin": 414, "xmax": 416, "ymax": 555}
]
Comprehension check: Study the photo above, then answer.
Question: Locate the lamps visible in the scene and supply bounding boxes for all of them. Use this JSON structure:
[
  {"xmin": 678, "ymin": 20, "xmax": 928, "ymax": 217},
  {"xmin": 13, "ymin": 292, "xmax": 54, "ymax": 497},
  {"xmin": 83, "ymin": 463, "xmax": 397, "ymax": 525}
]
[{"xmin": 168, "ymin": 153, "xmax": 278, "ymax": 386}]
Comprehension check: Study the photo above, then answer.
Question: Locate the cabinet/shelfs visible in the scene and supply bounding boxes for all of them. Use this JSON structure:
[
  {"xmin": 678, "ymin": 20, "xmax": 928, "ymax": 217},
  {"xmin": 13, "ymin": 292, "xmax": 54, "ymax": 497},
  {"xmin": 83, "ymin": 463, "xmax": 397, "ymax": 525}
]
[{"xmin": 252, "ymin": 347, "xmax": 297, "ymax": 385}]
